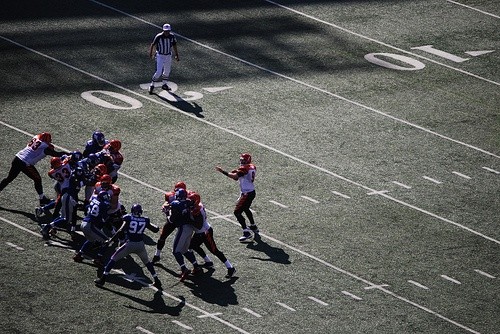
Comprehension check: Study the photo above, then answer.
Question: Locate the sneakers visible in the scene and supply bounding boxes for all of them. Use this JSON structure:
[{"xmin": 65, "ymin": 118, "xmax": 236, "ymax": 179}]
[
  {"xmin": 176, "ymin": 260, "xmax": 218, "ymax": 285},
  {"xmin": 249, "ymin": 226, "xmax": 260, "ymax": 234},
  {"xmin": 162, "ymin": 83, "xmax": 172, "ymax": 91},
  {"xmin": 93, "ymin": 276, "xmax": 105, "ymax": 285},
  {"xmin": 224, "ymin": 267, "xmax": 236, "ymax": 278},
  {"xmin": 39, "ymin": 197, "xmax": 55, "ymax": 207},
  {"xmin": 152, "ymin": 280, "xmax": 161, "ymax": 287},
  {"xmin": 40, "ymin": 229, "xmax": 50, "ymax": 239},
  {"xmin": 239, "ymin": 229, "xmax": 252, "ymax": 242},
  {"xmin": 72, "ymin": 251, "xmax": 82, "ymax": 261},
  {"xmin": 34, "ymin": 207, "xmax": 42, "ymax": 219},
  {"xmin": 149, "ymin": 255, "xmax": 160, "ymax": 265},
  {"xmin": 148, "ymin": 86, "xmax": 154, "ymax": 94}
]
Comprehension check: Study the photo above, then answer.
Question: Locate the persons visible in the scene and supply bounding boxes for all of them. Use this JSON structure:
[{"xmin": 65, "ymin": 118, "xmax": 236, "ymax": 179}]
[
  {"xmin": 34, "ymin": 130, "xmax": 237, "ymax": 294},
  {"xmin": 147, "ymin": 24, "xmax": 181, "ymax": 95},
  {"xmin": 216, "ymin": 152, "xmax": 259, "ymax": 242},
  {"xmin": 1, "ymin": 132, "xmax": 72, "ymax": 206}
]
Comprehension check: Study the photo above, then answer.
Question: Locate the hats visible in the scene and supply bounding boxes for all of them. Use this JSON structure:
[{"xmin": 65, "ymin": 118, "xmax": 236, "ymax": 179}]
[{"xmin": 163, "ymin": 24, "xmax": 171, "ymax": 31}]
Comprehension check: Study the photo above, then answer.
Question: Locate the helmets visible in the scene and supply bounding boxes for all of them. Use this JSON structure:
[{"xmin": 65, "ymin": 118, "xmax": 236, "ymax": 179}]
[
  {"xmin": 65, "ymin": 128, "xmax": 127, "ymax": 205},
  {"xmin": 239, "ymin": 153, "xmax": 252, "ymax": 165},
  {"xmin": 131, "ymin": 204, "xmax": 143, "ymax": 215},
  {"xmin": 39, "ymin": 132, "xmax": 52, "ymax": 144},
  {"xmin": 50, "ymin": 157, "xmax": 63, "ymax": 169},
  {"xmin": 164, "ymin": 181, "xmax": 206, "ymax": 221}
]
